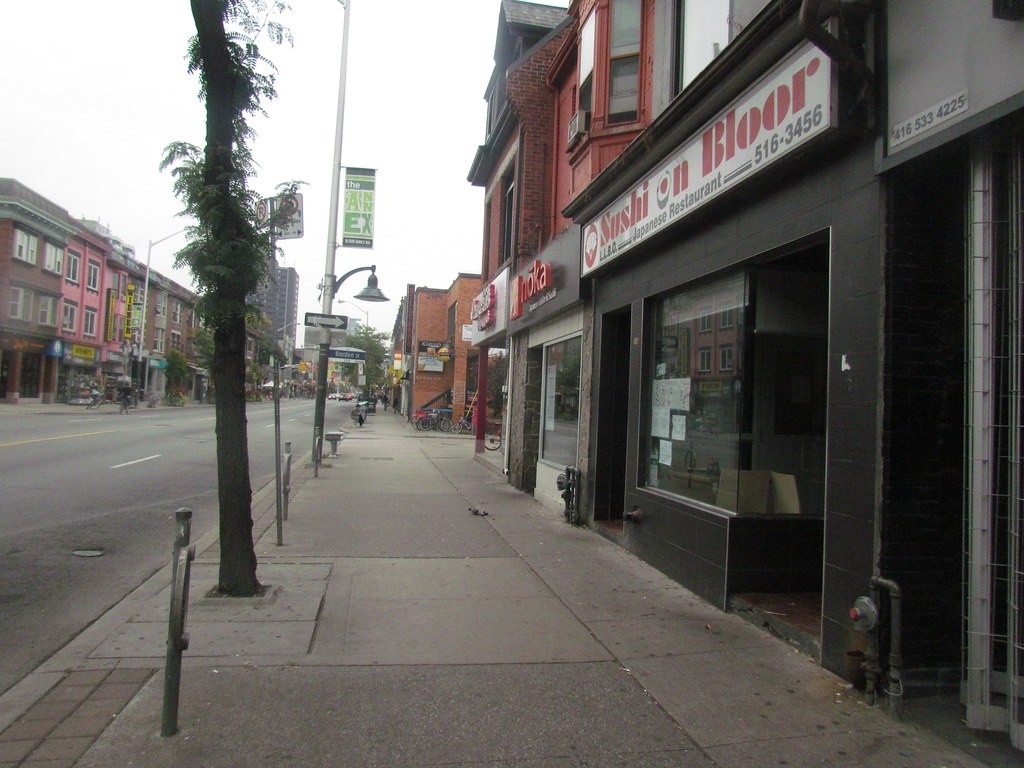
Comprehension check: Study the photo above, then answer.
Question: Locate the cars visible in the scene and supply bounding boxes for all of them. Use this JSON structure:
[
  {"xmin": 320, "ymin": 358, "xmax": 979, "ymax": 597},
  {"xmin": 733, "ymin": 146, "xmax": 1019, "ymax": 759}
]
[{"xmin": 328, "ymin": 391, "xmax": 363, "ymax": 401}]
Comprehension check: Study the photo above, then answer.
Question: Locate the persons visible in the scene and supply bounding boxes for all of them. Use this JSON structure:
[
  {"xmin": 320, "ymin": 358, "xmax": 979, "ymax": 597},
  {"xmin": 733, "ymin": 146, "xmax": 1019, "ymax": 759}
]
[
  {"xmin": 351, "ymin": 403, "xmax": 366, "ymax": 428},
  {"xmin": 121, "ymin": 383, "xmax": 132, "ymax": 414},
  {"xmin": 382, "ymin": 394, "xmax": 388, "ymax": 411}
]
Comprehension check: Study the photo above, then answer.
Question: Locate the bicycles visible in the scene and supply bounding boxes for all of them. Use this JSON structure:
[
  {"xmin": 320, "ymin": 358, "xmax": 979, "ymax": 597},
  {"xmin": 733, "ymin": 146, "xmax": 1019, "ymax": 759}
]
[
  {"xmin": 86, "ymin": 393, "xmax": 105, "ymax": 409},
  {"xmin": 410, "ymin": 408, "xmax": 472, "ymax": 435},
  {"xmin": 484, "ymin": 421, "xmax": 502, "ymax": 451},
  {"xmin": 119, "ymin": 397, "xmax": 131, "ymax": 415}
]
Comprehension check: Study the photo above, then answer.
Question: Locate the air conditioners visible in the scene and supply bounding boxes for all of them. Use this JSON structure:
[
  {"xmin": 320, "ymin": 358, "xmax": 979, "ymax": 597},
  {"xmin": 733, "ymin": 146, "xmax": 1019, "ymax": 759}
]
[{"xmin": 567, "ymin": 110, "xmax": 591, "ymax": 142}]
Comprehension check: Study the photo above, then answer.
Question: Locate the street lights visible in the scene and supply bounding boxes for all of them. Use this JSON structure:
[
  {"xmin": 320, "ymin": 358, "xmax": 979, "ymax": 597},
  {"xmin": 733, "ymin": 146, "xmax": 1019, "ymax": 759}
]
[
  {"xmin": 309, "ymin": 264, "xmax": 391, "ymax": 464},
  {"xmin": 134, "ymin": 226, "xmax": 197, "ymax": 407},
  {"xmin": 335, "ymin": 298, "xmax": 369, "ymax": 332}
]
